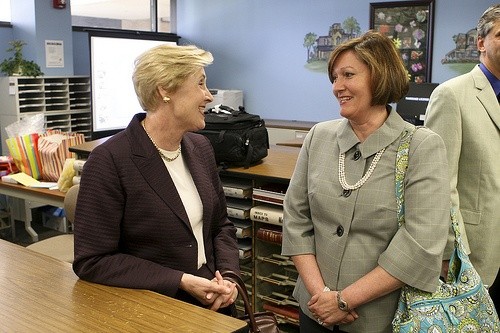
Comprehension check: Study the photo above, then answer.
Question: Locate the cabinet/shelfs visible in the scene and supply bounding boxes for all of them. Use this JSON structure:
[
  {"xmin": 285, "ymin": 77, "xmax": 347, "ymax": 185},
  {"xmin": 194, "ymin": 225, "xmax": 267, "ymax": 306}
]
[
  {"xmin": 68, "ymin": 127, "xmax": 311, "ymax": 333},
  {"xmin": 0, "ymin": 75, "xmax": 92, "ymax": 157}
]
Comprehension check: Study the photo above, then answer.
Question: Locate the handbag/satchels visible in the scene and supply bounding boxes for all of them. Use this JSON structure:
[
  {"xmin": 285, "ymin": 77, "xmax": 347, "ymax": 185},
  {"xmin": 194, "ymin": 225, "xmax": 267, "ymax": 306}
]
[
  {"xmin": 198, "ymin": 105, "xmax": 270, "ymax": 170},
  {"xmin": 391, "ymin": 248, "xmax": 500, "ymax": 333},
  {"xmin": 5, "ymin": 128, "xmax": 85, "ymax": 182}
]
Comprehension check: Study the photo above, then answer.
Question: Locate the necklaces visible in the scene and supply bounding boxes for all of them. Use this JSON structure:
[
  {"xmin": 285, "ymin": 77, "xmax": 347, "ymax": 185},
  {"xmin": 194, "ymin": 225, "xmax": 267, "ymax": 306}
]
[
  {"xmin": 338, "ymin": 148, "xmax": 386, "ymax": 190},
  {"xmin": 142, "ymin": 119, "xmax": 181, "ymax": 162}
]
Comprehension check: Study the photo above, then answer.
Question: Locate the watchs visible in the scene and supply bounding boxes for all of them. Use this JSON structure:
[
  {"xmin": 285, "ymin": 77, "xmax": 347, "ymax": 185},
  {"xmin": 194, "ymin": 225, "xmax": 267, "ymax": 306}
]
[{"xmin": 336, "ymin": 291, "xmax": 350, "ymax": 312}]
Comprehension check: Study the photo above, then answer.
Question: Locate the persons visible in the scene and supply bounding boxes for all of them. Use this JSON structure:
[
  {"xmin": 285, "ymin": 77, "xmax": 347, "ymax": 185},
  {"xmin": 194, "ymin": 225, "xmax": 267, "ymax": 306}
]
[
  {"xmin": 280, "ymin": 30, "xmax": 450, "ymax": 333},
  {"xmin": 424, "ymin": 4, "xmax": 500, "ymax": 321},
  {"xmin": 72, "ymin": 45, "xmax": 240, "ymax": 318}
]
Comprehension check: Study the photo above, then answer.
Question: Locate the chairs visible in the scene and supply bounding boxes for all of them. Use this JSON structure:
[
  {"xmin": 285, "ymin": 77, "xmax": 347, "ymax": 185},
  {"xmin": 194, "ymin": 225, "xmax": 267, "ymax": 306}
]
[{"xmin": 25, "ymin": 184, "xmax": 79, "ymax": 263}]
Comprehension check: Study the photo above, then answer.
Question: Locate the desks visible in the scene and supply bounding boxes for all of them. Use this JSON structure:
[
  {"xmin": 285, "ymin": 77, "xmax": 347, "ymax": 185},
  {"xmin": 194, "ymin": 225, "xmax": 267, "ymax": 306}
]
[
  {"xmin": 0, "ymin": 238, "xmax": 249, "ymax": 333},
  {"xmin": 0, "ymin": 178, "xmax": 69, "ymax": 242},
  {"xmin": 262, "ymin": 119, "xmax": 318, "ymax": 131}
]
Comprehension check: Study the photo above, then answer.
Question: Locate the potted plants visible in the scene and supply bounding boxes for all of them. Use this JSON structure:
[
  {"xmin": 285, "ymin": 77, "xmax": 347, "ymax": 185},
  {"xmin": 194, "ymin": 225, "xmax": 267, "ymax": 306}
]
[{"xmin": 0, "ymin": 40, "xmax": 44, "ymax": 78}]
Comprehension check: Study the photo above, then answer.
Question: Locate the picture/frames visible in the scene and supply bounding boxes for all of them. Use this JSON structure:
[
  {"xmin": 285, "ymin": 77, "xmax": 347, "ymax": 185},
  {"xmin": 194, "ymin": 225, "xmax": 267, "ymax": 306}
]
[{"xmin": 369, "ymin": 0, "xmax": 435, "ymax": 84}]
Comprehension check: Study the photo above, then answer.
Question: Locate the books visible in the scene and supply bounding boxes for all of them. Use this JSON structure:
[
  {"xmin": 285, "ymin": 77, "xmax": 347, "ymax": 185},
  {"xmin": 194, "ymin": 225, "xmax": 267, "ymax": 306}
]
[{"xmin": 220, "ymin": 182, "xmax": 299, "ymax": 326}]
